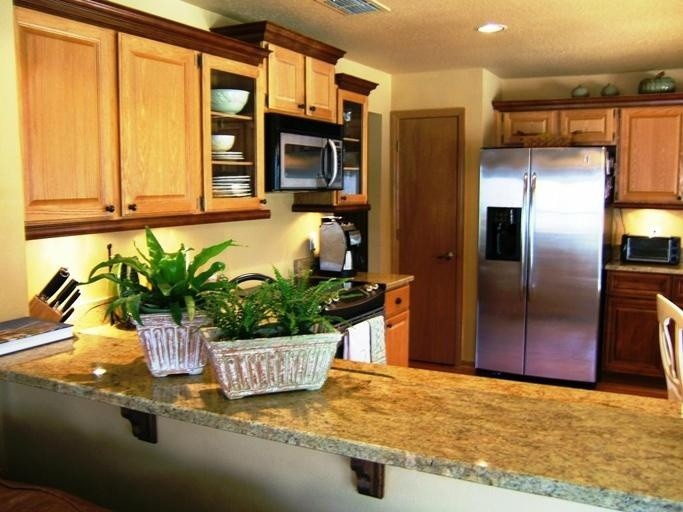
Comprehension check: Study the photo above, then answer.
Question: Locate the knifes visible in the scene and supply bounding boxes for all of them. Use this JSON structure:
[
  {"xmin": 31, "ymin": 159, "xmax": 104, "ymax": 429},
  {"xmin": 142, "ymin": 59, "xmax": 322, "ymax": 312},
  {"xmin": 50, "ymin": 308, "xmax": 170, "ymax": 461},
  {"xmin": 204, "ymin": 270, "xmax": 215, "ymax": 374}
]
[{"xmin": 38, "ymin": 266, "xmax": 81, "ymax": 321}]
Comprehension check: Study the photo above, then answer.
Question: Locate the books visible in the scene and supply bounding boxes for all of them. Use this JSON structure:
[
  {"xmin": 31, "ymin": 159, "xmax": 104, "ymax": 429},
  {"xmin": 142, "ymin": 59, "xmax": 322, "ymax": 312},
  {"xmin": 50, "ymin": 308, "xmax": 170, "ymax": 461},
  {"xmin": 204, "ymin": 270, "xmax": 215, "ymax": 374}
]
[{"xmin": 0, "ymin": 316, "xmax": 75, "ymax": 357}]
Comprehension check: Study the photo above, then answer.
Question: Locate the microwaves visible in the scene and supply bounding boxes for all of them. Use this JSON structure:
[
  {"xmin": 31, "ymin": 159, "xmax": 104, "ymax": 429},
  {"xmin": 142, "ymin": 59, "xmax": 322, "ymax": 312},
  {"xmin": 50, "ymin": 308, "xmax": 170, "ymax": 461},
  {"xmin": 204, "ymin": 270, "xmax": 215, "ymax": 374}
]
[{"xmin": 265, "ymin": 129, "xmax": 344, "ymax": 190}]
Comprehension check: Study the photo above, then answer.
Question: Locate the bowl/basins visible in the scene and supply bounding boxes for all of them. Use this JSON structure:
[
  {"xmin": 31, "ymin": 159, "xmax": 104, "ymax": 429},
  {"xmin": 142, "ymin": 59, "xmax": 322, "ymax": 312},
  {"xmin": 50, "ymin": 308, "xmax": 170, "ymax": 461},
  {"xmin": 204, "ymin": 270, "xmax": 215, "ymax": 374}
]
[
  {"xmin": 212, "ymin": 135, "xmax": 235, "ymax": 151},
  {"xmin": 212, "ymin": 87, "xmax": 251, "ymax": 114}
]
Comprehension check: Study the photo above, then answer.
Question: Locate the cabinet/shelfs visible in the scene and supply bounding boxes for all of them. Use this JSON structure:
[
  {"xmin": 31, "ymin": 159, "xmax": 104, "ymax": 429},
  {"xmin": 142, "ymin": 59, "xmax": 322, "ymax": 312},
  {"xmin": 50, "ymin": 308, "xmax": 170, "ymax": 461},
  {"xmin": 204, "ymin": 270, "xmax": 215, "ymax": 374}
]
[
  {"xmin": 618, "ymin": 106, "xmax": 683, "ymax": 205},
  {"xmin": 202, "ymin": 51, "xmax": 264, "ymax": 213},
  {"xmin": 559, "ymin": 108, "xmax": 614, "ymax": 143},
  {"xmin": 267, "ymin": 43, "xmax": 335, "ymax": 120},
  {"xmin": 602, "ymin": 272, "xmax": 671, "ymax": 377},
  {"xmin": 294, "ymin": 88, "xmax": 368, "ymax": 206},
  {"xmin": 13, "ymin": 4, "xmax": 202, "ymax": 227},
  {"xmin": 502, "ymin": 110, "xmax": 558, "ymax": 144},
  {"xmin": 384, "ymin": 285, "xmax": 410, "ymax": 367}
]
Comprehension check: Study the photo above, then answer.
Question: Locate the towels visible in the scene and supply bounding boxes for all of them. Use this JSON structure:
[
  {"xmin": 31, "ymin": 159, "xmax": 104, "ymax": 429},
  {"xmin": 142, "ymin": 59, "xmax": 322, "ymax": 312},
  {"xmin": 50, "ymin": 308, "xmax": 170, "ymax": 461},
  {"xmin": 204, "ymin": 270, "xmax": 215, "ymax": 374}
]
[
  {"xmin": 368, "ymin": 315, "xmax": 386, "ymax": 365},
  {"xmin": 343, "ymin": 322, "xmax": 372, "ymax": 363}
]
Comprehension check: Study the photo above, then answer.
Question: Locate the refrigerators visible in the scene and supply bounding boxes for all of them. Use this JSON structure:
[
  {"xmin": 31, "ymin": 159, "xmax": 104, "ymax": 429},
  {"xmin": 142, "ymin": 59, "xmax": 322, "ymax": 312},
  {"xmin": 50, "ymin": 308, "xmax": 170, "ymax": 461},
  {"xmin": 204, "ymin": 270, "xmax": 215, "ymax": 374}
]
[{"xmin": 474, "ymin": 148, "xmax": 612, "ymax": 388}]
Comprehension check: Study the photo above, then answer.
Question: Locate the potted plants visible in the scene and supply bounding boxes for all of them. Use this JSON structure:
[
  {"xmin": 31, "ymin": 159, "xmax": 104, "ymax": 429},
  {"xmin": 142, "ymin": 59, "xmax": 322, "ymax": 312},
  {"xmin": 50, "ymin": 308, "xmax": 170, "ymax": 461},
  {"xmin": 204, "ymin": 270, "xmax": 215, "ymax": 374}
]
[
  {"xmin": 200, "ymin": 265, "xmax": 368, "ymax": 400},
  {"xmin": 76, "ymin": 225, "xmax": 236, "ymax": 378}
]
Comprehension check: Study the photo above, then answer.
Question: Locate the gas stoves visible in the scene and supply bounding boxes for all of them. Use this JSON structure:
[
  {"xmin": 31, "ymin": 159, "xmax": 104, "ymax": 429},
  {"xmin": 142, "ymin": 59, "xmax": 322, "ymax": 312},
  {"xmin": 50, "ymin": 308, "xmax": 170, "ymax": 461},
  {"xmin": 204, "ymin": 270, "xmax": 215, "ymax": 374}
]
[{"xmin": 235, "ymin": 277, "xmax": 386, "ymax": 314}]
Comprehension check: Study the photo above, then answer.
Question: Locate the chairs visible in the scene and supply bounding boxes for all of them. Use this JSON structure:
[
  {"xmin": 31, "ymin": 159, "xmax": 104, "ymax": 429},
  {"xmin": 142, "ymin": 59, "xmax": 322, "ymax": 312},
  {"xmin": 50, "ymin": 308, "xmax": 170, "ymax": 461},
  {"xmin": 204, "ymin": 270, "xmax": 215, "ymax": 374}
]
[{"xmin": 656, "ymin": 294, "xmax": 683, "ymax": 401}]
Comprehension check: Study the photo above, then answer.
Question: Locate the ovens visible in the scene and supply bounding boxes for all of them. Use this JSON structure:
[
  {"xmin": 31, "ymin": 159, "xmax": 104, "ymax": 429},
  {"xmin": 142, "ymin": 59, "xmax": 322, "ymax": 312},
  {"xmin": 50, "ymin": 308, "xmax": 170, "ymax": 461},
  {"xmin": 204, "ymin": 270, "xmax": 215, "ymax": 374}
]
[
  {"xmin": 620, "ymin": 234, "xmax": 680, "ymax": 266},
  {"xmin": 327, "ymin": 308, "xmax": 386, "ymax": 365}
]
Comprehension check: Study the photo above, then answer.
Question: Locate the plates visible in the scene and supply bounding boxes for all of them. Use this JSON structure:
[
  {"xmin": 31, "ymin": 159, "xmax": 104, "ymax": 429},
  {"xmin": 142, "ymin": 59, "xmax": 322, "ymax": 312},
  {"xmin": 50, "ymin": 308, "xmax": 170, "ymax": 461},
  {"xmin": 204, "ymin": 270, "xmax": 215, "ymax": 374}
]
[
  {"xmin": 212, "ymin": 175, "xmax": 253, "ymax": 198},
  {"xmin": 213, "ymin": 151, "xmax": 246, "ymax": 161}
]
